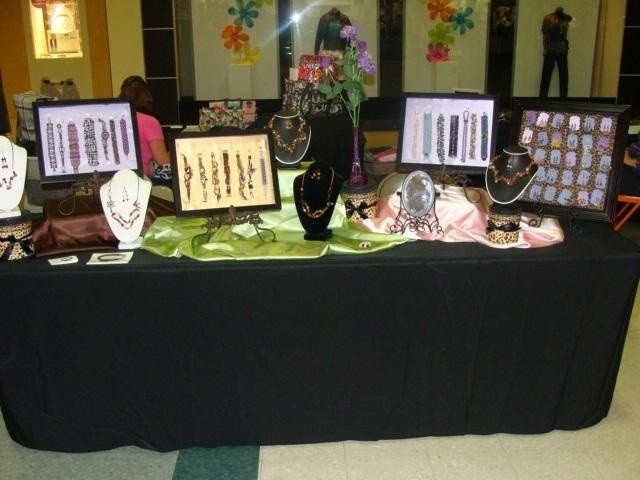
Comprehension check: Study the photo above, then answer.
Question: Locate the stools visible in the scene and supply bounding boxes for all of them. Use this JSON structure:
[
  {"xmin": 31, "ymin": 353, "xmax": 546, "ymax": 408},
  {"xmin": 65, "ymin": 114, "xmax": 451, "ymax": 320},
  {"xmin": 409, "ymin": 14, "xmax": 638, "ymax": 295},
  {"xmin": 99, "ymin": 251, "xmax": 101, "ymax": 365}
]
[{"xmin": 611, "ymin": 196, "xmax": 639, "ymax": 237}]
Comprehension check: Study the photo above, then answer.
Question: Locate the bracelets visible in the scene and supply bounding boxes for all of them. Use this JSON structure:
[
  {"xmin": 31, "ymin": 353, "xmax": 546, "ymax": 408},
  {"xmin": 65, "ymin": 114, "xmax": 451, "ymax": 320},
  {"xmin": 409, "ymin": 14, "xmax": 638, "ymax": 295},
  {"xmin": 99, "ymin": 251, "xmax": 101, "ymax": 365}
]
[
  {"xmin": 181, "ymin": 147, "xmax": 267, "ymax": 203},
  {"xmin": 45, "ymin": 114, "xmax": 130, "ymax": 175},
  {"xmin": 410, "ymin": 110, "xmax": 488, "ymax": 164}
]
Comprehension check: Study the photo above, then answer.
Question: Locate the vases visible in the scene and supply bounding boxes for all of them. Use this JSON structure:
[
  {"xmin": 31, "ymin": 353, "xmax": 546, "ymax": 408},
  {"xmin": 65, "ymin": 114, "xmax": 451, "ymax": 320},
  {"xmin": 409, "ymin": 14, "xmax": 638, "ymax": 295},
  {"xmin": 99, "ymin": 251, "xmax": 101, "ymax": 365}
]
[{"xmin": 350, "ymin": 125, "xmax": 366, "ymax": 186}]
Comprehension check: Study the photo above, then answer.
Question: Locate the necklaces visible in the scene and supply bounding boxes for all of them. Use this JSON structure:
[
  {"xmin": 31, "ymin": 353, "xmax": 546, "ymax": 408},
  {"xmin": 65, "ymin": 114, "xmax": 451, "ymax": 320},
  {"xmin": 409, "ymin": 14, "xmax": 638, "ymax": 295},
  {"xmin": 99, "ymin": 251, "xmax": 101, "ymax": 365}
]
[
  {"xmin": 106, "ymin": 175, "xmax": 144, "ymax": 231},
  {"xmin": 488, "ymin": 154, "xmax": 536, "ymax": 185},
  {"xmin": 0, "ymin": 139, "xmax": 19, "ymax": 191},
  {"xmin": 268, "ymin": 117, "xmax": 310, "ymax": 155},
  {"xmin": 299, "ymin": 165, "xmax": 335, "ymax": 220}
]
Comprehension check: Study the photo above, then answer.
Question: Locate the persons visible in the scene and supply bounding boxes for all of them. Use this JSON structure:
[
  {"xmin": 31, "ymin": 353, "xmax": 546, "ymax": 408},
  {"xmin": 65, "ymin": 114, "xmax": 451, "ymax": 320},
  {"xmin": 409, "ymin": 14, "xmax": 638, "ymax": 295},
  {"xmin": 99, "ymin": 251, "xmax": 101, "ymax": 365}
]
[
  {"xmin": 115, "ymin": 74, "xmax": 177, "ymax": 179},
  {"xmin": 313, "ymin": 7, "xmax": 353, "ymax": 62},
  {"xmin": 538, "ymin": 4, "xmax": 573, "ymax": 98}
]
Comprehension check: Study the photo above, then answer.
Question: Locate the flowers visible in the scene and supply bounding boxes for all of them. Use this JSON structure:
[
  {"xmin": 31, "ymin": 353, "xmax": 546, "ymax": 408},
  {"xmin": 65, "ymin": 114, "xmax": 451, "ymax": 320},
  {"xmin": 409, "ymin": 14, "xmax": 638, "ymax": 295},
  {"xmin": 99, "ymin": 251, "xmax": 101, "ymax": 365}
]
[{"xmin": 315, "ymin": 26, "xmax": 377, "ymax": 128}]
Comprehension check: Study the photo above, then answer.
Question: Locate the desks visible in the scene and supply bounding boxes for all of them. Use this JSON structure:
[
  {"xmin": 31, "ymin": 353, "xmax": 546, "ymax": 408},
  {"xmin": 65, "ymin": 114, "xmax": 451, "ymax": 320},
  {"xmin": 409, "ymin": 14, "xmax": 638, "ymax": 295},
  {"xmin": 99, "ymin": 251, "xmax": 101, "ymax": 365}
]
[{"xmin": 0, "ymin": 197, "xmax": 639, "ymax": 450}]
